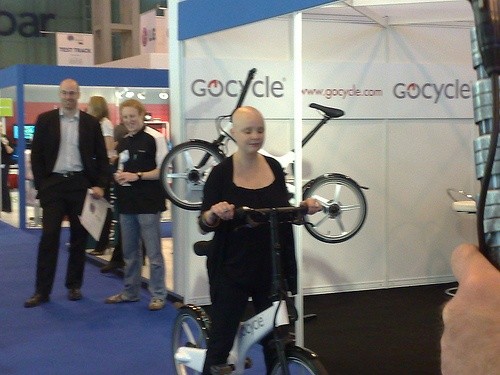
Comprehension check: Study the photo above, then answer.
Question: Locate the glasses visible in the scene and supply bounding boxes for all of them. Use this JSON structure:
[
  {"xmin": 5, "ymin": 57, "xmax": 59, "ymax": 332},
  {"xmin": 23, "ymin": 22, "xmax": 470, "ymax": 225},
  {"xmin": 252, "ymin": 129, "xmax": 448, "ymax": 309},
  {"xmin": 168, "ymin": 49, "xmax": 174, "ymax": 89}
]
[{"xmin": 60, "ymin": 90, "xmax": 79, "ymax": 95}]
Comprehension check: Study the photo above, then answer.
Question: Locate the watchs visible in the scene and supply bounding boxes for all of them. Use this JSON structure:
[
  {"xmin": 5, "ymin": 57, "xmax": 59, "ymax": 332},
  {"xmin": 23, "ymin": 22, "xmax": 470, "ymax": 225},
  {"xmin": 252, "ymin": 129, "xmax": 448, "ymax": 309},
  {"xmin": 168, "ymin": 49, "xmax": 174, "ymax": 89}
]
[{"xmin": 136, "ymin": 171, "xmax": 143, "ymax": 181}]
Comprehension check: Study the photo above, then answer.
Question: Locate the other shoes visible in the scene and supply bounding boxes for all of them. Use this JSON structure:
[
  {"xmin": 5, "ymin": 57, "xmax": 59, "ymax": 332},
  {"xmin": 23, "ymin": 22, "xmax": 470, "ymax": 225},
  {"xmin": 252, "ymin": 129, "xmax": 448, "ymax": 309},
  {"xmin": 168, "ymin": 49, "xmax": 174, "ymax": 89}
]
[
  {"xmin": 100, "ymin": 261, "xmax": 124, "ymax": 272},
  {"xmin": 104, "ymin": 293, "xmax": 140, "ymax": 303},
  {"xmin": 86, "ymin": 248, "xmax": 105, "ymax": 255},
  {"xmin": 148, "ymin": 295, "xmax": 165, "ymax": 309},
  {"xmin": 24, "ymin": 294, "xmax": 49, "ymax": 305},
  {"xmin": 70, "ymin": 287, "xmax": 81, "ymax": 300}
]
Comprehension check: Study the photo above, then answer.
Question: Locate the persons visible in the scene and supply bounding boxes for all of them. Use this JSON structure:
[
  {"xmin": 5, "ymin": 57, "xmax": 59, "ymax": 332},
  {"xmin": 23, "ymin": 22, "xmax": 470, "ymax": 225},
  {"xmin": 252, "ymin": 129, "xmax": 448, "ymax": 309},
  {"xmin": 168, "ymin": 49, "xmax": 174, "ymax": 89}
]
[
  {"xmin": 0, "ymin": 134, "xmax": 15, "ymax": 213},
  {"xmin": 103, "ymin": 99, "xmax": 171, "ymax": 310},
  {"xmin": 24, "ymin": 78, "xmax": 112, "ymax": 308},
  {"xmin": 82, "ymin": 96, "xmax": 146, "ymax": 275},
  {"xmin": 198, "ymin": 105, "xmax": 323, "ymax": 375}
]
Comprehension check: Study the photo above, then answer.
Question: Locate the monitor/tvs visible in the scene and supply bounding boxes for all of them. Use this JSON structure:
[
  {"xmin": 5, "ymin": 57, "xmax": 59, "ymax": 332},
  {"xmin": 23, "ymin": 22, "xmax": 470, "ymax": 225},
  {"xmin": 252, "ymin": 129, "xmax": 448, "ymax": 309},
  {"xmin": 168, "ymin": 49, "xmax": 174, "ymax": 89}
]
[{"xmin": 13, "ymin": 124, "xmax": 36, "ymax": 141}]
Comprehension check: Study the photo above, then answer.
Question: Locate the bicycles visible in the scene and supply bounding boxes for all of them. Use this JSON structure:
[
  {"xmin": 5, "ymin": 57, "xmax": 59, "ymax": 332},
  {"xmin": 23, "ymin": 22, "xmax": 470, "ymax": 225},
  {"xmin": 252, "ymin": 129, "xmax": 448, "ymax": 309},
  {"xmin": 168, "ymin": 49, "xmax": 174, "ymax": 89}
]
[
  {"xmin": 159, "ymin": 68, "xmax": 370, "ymax": 244},
  {"xmin": 170, "ymin": 206, "xmax": 328, "ymax": 375}
]
